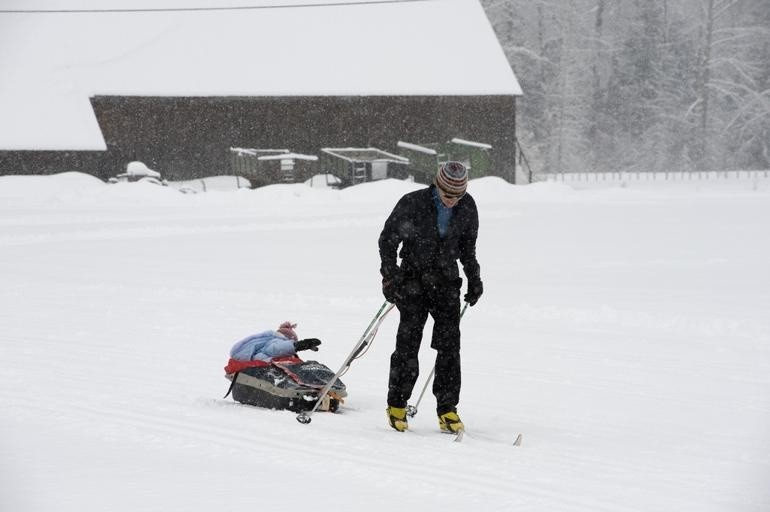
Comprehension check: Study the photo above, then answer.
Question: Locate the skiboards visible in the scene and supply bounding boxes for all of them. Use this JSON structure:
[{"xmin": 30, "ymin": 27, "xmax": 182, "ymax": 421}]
[{"xmin": 341, "ymin": 407, "xmax": 522, "ymax": 447}]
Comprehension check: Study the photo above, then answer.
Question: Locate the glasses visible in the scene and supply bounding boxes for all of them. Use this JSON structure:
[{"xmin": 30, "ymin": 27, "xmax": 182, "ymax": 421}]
[{"xmin": 443, "ymin": 193, "xmax": 464, "ymax": 200}]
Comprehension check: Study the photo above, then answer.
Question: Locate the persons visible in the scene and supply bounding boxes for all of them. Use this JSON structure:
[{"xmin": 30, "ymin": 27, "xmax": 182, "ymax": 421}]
[
  {"xmin": 378, "ymin": 160, "xmax": 484, "ymax": 433},
  {"xmin": 230, "ymin": 322, "xmax": 322, "ymax": 363}
]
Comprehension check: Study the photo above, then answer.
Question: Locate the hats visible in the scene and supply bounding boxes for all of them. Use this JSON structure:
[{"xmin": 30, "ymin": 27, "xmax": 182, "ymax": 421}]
[
  {"xmin": 438, "ymin": 162, "xmax": 468, "ymax": 195},
  {"xmin": 277, "ymin": 322, "xmax": 297, "ymax": 340}
]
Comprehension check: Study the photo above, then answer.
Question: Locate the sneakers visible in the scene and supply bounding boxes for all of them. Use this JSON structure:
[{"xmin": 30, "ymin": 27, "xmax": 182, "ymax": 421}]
[
  {"xmin": 388, "ymin": 407, "xmax": 408, "ymax": 430},
  {"xmin": 438, "ymin": 412, "xmax": 464, "ymax": 434}
]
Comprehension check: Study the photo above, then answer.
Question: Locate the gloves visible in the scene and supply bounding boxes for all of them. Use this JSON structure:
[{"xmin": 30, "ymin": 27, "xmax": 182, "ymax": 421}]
[
  {"xmin": 295, "ymin": 338, "xmax": 321, "ymax": 352},
  {"xmin": 464, "ymin": 279, "xmax": 483, "ymax": 305},
  {"xmin": 381, "ymin": 275, "xmax": 399, "ymax": 303}
]
